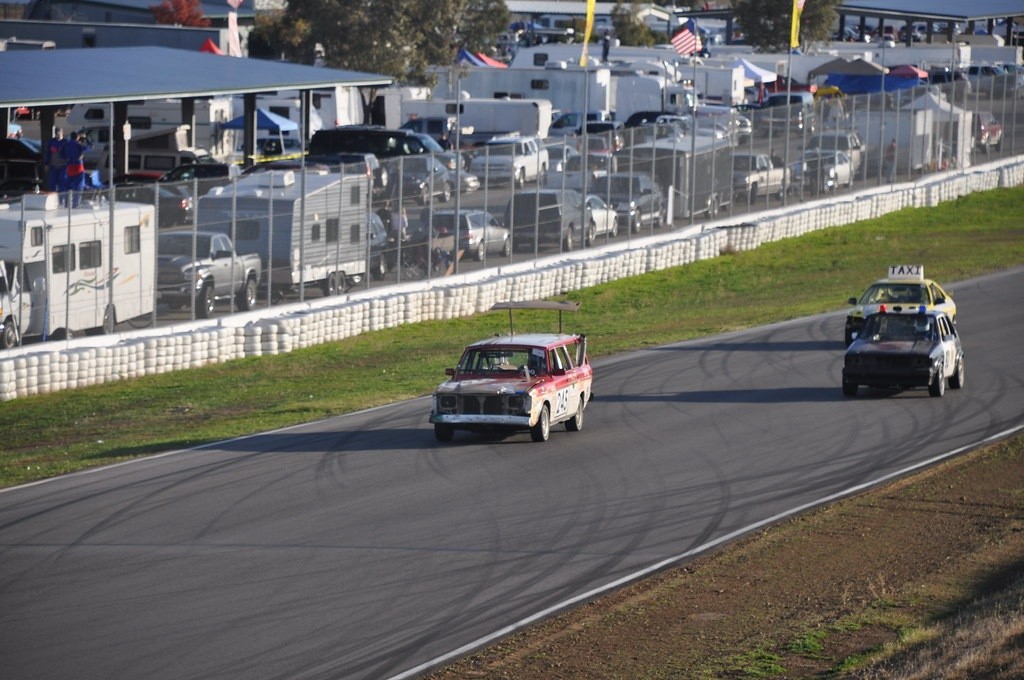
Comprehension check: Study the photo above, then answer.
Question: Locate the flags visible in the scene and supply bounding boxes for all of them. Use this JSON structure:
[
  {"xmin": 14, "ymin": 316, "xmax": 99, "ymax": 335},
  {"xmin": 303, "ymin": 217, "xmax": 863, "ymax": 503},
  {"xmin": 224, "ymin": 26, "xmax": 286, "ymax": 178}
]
[{"xmin": 670, "ymin": 16, "xmax": 704, "ymax": 57}]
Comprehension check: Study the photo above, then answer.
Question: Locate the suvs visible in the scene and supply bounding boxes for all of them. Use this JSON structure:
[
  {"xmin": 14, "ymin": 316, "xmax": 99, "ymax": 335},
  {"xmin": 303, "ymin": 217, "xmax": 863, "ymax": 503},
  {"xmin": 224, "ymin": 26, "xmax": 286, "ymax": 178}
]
[{"xmin": 429, "ymin": 301, "xmax": 593, "ymax": 442}]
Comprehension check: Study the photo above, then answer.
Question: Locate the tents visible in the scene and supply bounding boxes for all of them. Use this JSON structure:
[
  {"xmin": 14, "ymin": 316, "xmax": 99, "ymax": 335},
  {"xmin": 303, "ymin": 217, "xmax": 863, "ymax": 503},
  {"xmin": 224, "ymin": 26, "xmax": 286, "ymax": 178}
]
[
  {"xmin": 805, "ymin": 56, "xmax": 930, "ymax": 100},
  {"xmin": 220, "ymin": 106, "xmax": 304, "ymax": 157}
]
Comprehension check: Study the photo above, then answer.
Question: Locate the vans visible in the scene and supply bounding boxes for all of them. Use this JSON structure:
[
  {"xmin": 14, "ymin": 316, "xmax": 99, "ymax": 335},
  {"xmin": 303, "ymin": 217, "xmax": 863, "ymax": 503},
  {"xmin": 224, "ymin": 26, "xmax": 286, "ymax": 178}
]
[
  {"xmin": 616, "ymin": 123, "xmax": 736, "ymax": 220},
  {"xmin": 945, "ymin": 109, "xmax": 1006, "ymax": 155}
]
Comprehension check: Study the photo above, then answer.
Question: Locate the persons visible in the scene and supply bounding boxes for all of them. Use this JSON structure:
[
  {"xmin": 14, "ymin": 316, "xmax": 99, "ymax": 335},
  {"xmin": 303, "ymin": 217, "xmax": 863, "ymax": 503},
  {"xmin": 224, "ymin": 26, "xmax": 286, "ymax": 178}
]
[
  {"xmin": 847, "ymin": 22, "xmax": 861, "ymax": 43},
  {"xmin": 601, "ymin": 29, "xmax": 610, "ymax": 62},
  {"xmin": 905, "ymin": 287, "xmax": 923, "ymax": 302},
  {"xmin": 44, "ymin": 126, "xmax": 94, "ymax": 207},
  {"xmin": 914, "ymin": 316, "xmax": 938, "ymax": 341},
  {"xmin": 376, "ymin": 201, "xmax": 438, "ymax": 277},
  {"xmin": 769, "ymin": 148, "xmax": 785, "ymax": 168},
  {"xmin": 518, "ymin": 349, "xmax": 554, "ymax": 372},
  {"xmin": 884, "ymin": 138, "xmax": 898, "ymax": 183}
]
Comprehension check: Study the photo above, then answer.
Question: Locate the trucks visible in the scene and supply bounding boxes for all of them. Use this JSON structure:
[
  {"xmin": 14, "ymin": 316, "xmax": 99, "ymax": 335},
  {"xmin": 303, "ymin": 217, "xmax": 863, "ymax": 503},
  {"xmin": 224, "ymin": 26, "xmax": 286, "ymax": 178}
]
[{"xmin": 0, "ymin": 191, "xmax": 157, "ymax": 350}]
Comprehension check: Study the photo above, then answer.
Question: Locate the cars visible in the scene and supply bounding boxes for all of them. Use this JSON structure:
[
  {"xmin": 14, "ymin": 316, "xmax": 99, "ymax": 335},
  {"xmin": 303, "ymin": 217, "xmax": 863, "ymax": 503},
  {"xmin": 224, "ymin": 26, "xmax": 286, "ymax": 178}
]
[
  {"xmin": 580, "ymin": 193, "xmax": 619, "ymax": 238},
  {"xmin": 801, "ymin": 130, "xmax": 870, "ymax": 177},
  {"xmin": 425, "ymin": 207, "xmax": 512, "ymax": 260},
  {"xmin": 731, "ymin": 151, "xmax": 794, "ymax": 205},
  {"xmin": 800, "ymin": 147, "xmax": 856, "ymax": 197},
  {"xmin": 0, "ymin": 20, "xmax": 1024, "ymax": 222},
  {"xmin": 844, "ymin": 264, "xmax": 957, "ymax": 346},
  {"xmin": 590, "ymin": 172, "xmax": 666, "ymax": 234},
  {"xmin": 368, "ymin": 211, "xmax": 391, "ymax": 282},
  {"xmin": 840, "ymin": 302, "xmax": 966, "ymax": 397},
  {"xmin": 503, "ymin": 189, "xmax": 596, "ymax": 253}
]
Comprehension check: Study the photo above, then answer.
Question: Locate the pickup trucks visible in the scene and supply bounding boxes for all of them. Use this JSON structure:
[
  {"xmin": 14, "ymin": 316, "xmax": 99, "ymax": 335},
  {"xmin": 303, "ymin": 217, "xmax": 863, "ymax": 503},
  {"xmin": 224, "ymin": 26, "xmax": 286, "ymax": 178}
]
[{"xmin": 155, "ymin": 229, "xmax": 263, "ymax": 319}]
